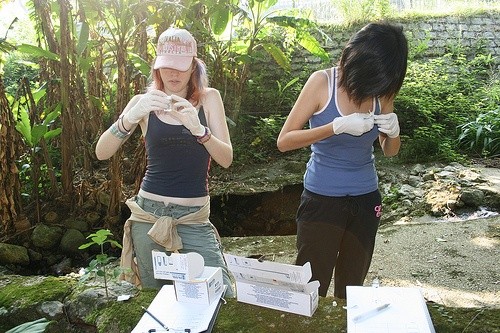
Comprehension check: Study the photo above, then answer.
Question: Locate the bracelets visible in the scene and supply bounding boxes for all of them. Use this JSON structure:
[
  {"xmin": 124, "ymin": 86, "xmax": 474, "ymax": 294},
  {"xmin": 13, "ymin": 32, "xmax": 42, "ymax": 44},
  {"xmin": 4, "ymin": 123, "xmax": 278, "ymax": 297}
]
[
  {"xmin": 111, "ymin": 115, "xmax": 131, "ymax": 138},
  {"xmin": 196, "ymin": 127, "xmax": 211, "ymax": 145}
]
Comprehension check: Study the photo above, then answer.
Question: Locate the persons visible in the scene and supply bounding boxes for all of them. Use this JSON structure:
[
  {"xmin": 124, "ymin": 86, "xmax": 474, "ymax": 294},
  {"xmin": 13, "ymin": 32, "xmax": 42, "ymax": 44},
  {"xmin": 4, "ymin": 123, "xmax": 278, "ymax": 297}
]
[
  {"xmin": 277, "ymin": 23, "xmax": 409, "ymax": 300},
  {"xmin": 95, "ymin": 27, "xmax": 234, "ymax": 298}
]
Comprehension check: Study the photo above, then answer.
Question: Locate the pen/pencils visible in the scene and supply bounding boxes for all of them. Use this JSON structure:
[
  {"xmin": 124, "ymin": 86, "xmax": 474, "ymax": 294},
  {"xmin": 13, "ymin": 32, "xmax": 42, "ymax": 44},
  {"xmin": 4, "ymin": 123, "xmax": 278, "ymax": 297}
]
[
  {"xmin": 354, "ymin": 303, "xmax": 390, "ymax": 320},
  {"xmin": 141, "ymin": 305, "xmax": 168, "ymax": 329}
]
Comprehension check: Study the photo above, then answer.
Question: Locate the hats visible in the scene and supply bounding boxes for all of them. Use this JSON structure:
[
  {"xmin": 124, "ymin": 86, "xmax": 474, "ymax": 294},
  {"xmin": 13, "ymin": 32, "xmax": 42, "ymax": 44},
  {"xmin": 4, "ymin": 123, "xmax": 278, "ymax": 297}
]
[{"xmin": 153, "ymin": 28, "xmax": 197, "ymax": 71}]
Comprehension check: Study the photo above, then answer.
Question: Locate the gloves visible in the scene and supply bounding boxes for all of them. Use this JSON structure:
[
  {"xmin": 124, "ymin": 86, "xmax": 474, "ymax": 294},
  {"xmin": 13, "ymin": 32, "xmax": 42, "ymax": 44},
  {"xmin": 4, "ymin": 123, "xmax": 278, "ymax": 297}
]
[
  {"xmin": 333, "ymin": 113, "xmax": 374, "ymax": 136},
  {"xmin": 371, "ymin": 113, "xmax": 400, "ymax": 138},
  {"xmin": 127, "ymin": 89, "xmax": 171, "ymax": 124},
  {"xmin": 169, "ymin": 94, "xmax": 205, "ymax": 137}
]
